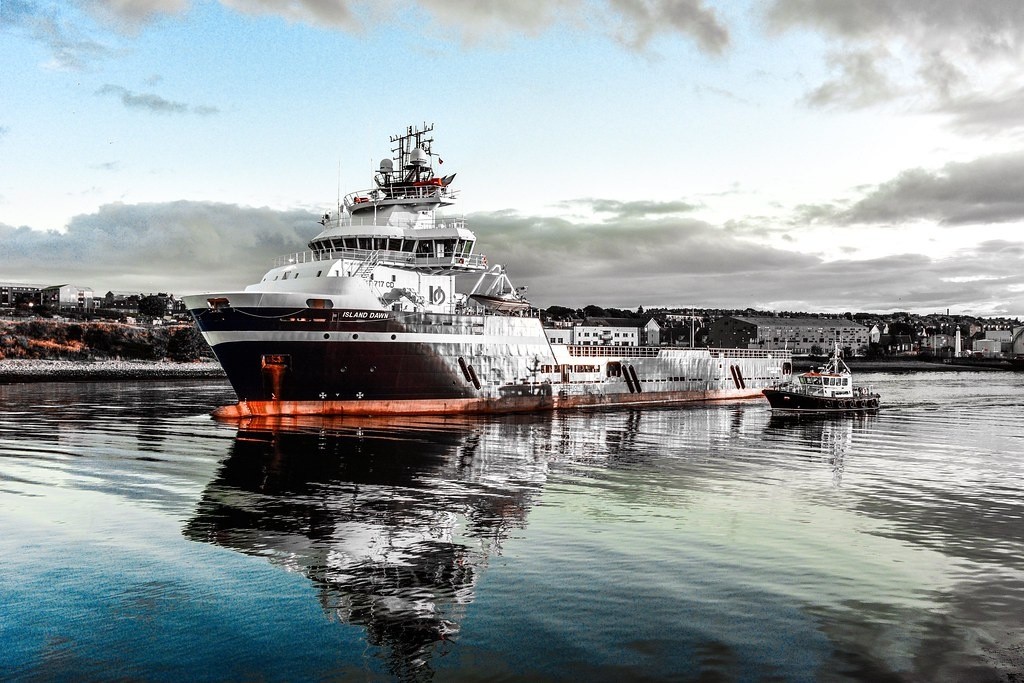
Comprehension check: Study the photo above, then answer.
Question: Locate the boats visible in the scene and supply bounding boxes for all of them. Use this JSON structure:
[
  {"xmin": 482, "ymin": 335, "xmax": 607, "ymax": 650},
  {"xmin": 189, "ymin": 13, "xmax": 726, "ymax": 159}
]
[
  {"xmin": 180, "ymin": 120, "xmax": 794, "ymax": 420},
  {"xmin": 761, "ymin": 342, "xmax": 881, "ymax": 411}
]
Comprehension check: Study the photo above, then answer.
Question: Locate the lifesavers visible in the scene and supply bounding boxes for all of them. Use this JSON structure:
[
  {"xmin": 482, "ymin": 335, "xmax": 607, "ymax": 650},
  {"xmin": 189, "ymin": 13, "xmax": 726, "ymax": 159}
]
[
  {"xmin": 871, "ymin": 401, "xmax": 875, "ymax": 407},
  {"xmin": 825, "ymin": 401, "xmax": 833, "ymax": 408},
  {"xmin": 861, "ymin": 402, "xmax": 865, "ymax": 407},
  {"xmin": 459, "ymin": 259, "xmax": 463, "ymax": 263},
  {"xmin": 862, "ymin": 388, "xmax": 867, "ymax": 394},
  {"xmin": 850, "ymin": 401, "xmax": 854, "ymax": 407},
  {"xmin": 484, "ymin": 257, "xmax": 486, "ymax": 263},
  {"xmin": 838, "ymin": 401, "xmax": 845, "ymax": 408}
]
